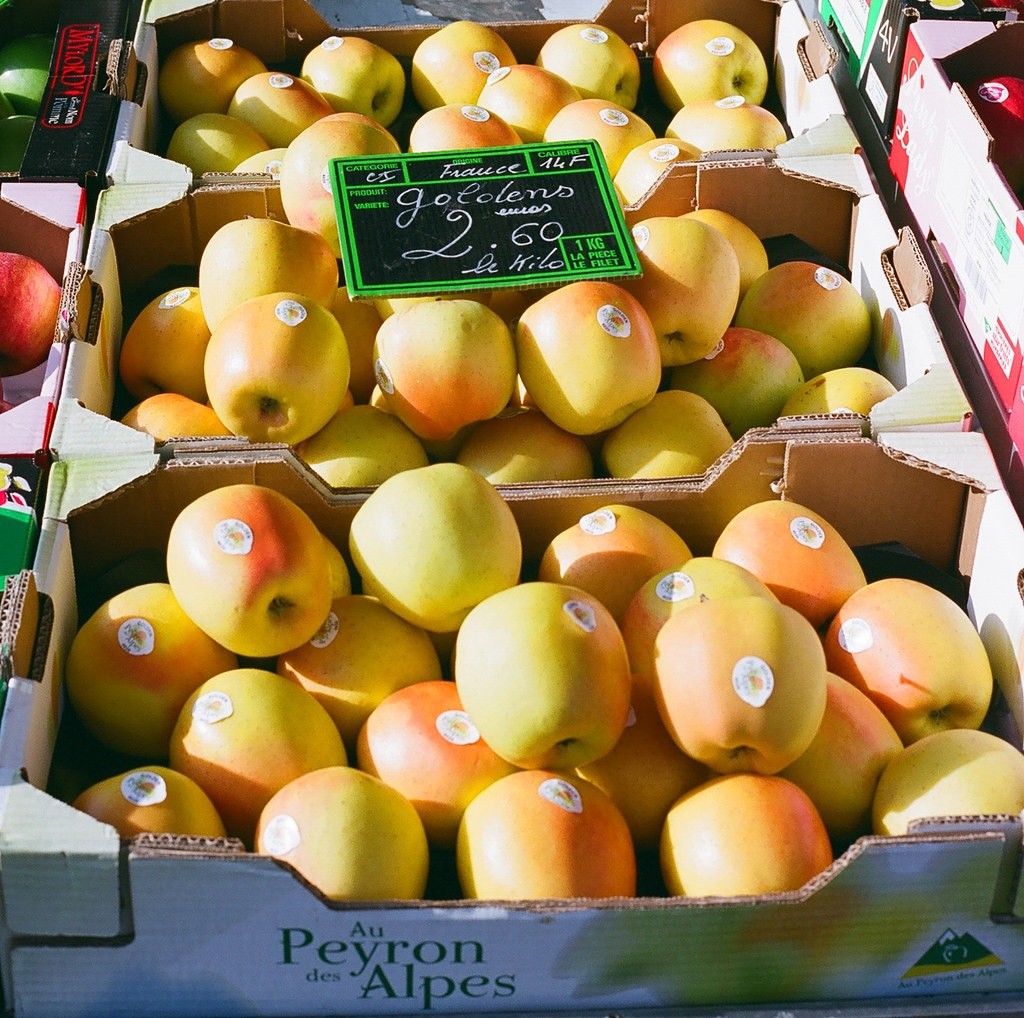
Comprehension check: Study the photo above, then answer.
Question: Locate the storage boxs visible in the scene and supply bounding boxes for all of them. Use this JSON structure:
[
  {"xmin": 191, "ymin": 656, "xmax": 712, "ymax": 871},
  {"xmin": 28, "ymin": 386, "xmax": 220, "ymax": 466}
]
[{"xmin": 0, "ymin": 0, "xmax": 1024, "ymax": 1018}]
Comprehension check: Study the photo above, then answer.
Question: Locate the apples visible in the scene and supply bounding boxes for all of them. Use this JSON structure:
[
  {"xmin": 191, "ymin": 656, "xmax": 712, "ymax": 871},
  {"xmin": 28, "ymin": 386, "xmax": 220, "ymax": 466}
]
[
  {"xmin": 0, "ymin": 249, "xmax": 64, "ymax": 376},
  {"xmin": 119, "ymin": 113, "xmax": 905, "ymax": 487},
  {"xmin": 63, "ymin": 460, "xmax": 1024, "ymax": 907},
  {"xmin": 0, "ymin": 0, "xmax": 56, "ymax": 173},
  {"xmin": 159, "ymin": 20, "xmax": 789, "ymax": 200},
  {"xmin": 965, "ymin": 76, "xmax": 1024, "ymax": 199}
]
